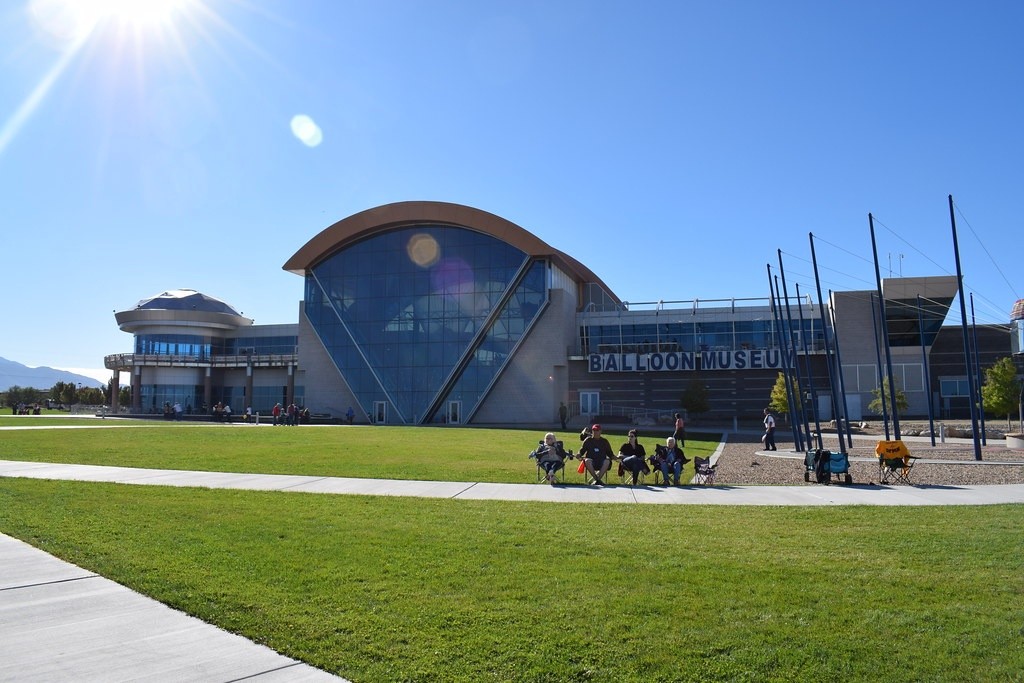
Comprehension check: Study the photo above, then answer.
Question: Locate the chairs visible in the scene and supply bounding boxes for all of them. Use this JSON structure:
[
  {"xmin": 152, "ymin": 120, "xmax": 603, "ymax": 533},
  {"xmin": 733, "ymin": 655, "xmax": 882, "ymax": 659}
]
[
  {"xmin": 649, "ymin": 443, "xmax": 684, "ymax": 485},
  {"xmin": 535, "ymin": 441, "xmax": 573, "ymax": 483},
  {"xmin": 694, "ymin": 456, "xmax": 718, "ymax": 486},
  {"xmin": 579, "ymin": 456, "xmax": 612, "ymax": 484},
  {"xmin": 617, "ymin": 454, "xmax": 644, "ymax": 486},
  {"xmin": 875, "ymin": 440, "xmax": 921, "ymax": 485}
]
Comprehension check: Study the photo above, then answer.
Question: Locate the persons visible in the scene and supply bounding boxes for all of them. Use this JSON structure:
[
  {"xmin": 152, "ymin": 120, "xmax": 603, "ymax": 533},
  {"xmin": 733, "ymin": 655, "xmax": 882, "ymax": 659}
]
[
  {"xmin": 672, "ymin": 413, "xmax": 685, "ymax": 447},
  {"xmin": 273, "ymin": 403, "xmax": 310, "ymax": 426},
  {"xmin": 163, "ymin": 402, "xmax": 183, "ymax": 421},
  {"xmin": 618, "ymin": 430, "xmax": 650, "ymax": 486},
  {"xmin": 558, "ymin": 402, "xmax": 567, "ymax": 429},
  {"xmin": 244, "ymin": 404, "xmax": 252, "ymax": 423},
  {"xmin": 213, "ymin": 401, "xmax": 232, "ymax": 422},
  {"xmin": 536, "ymin": 433, "xmax": 566, "ymax": 485},
  {"xmin": 763, "ymin": 408, "xmax": 776, "ymax": 451},
  {"xmin": 655, "ymin": 437, "xmax": 686, "ymax": 486},
  {"xmin": 576, "ymin": 424, "xmax": 618, "ymax": 486}
]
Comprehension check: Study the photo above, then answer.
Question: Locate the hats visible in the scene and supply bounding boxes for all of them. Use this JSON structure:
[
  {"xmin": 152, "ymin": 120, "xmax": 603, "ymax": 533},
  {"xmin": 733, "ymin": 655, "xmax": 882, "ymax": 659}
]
[{"xmin": 592, "ymin": 424, "xmax": 601, "ymax": 431}]
